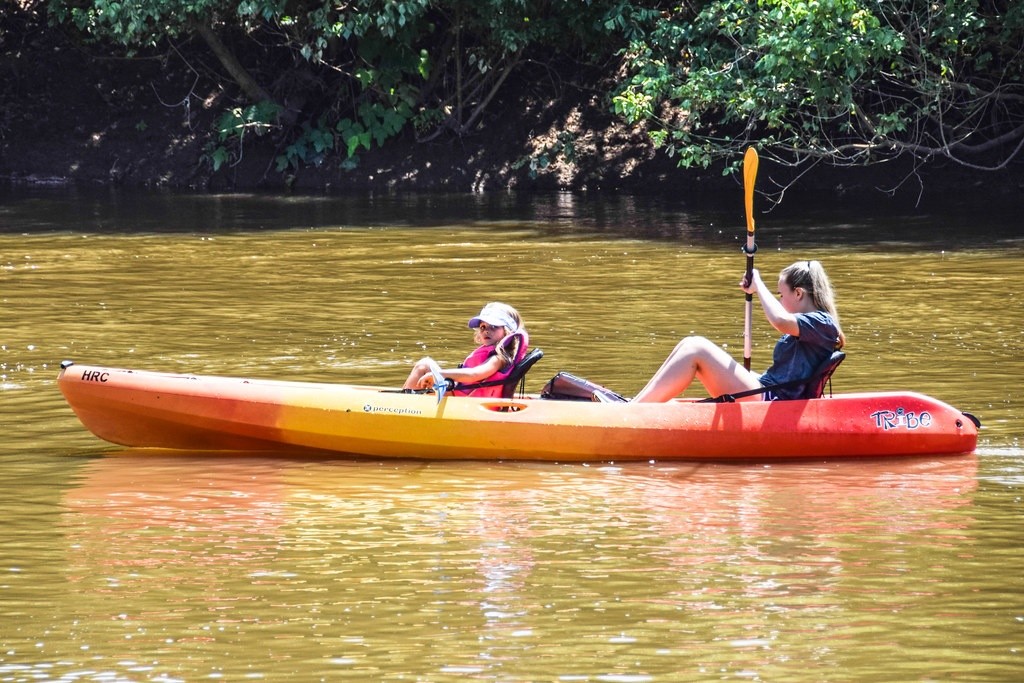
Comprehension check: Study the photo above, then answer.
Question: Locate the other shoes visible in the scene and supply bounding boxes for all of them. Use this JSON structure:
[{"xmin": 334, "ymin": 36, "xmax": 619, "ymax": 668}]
[{"xmin": 591, "ymin": 390, "xmax": 613, "ymax": 402}]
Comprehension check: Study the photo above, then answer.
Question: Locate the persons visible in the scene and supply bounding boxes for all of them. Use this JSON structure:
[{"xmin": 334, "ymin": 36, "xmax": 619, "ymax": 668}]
[
  {"xmin": 402, "ymin": 302, "xmax": 529, "ymax": 398},
  {"xmin": 591, "ymin": 261, "xmax": 845, "ymax": 404}
]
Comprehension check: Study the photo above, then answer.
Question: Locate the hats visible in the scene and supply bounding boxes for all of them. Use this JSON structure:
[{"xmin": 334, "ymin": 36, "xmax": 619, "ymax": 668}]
[{"xmin": 469, "ymin": 308, "xmax": 517, "ymax": 331}]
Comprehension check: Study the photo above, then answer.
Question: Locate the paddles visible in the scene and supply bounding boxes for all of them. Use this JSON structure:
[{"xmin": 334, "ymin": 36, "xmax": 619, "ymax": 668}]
[
  {"xmin": 741, "ymin": 142, "xmax": 761, "ymax": 377},
  {"xmin": 427, "ymin": 364, "xmax": 458, "ymax": 404}
]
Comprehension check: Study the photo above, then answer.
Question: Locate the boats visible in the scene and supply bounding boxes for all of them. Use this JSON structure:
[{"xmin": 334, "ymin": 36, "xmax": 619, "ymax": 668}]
[{"xmin": 58, "ymin": 349, "xmax": 981, "ymax": 460}]
